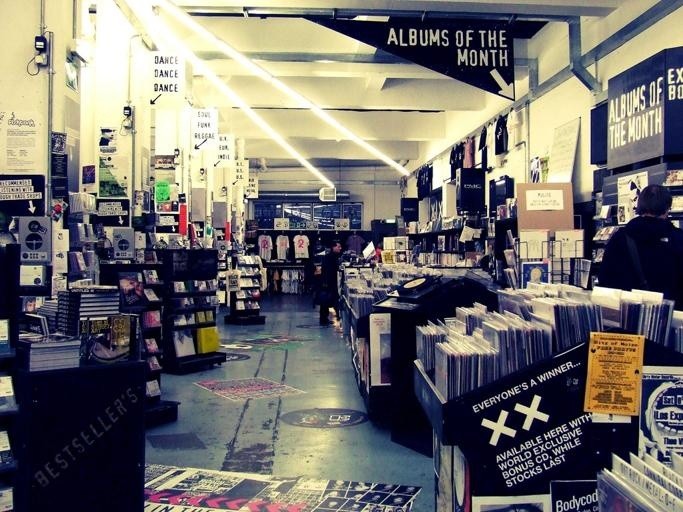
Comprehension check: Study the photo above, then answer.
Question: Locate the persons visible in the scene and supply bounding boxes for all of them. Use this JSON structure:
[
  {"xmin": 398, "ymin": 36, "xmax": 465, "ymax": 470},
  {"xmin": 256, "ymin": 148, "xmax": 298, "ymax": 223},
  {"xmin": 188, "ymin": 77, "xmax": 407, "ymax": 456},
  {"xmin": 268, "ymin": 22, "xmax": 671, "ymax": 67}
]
[
  {"xmin": 630, "ymin": 181, "xmax": 640, "ymax": 214},
  {"xmin": 598, "ymin": 184, "xmax": 683, "ymax": 311},
  {"xmin": 319, "ymin": 239, "xmax": 342, "ymax": 326}
]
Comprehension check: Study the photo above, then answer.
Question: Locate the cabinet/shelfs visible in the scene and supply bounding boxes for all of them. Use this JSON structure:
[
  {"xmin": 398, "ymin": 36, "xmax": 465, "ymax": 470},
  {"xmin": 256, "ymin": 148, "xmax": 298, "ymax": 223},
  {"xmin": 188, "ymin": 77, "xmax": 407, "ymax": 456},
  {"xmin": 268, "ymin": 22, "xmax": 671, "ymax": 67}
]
[
  {"xmin": 224, "ymin": 264, "xmax": 267, "ymax": 326},
  {"xmin": 99, "ymin": 263, "xmax": 181, "ymax": 429},
  {"xmin": 257, "ymin": 229, "xmax": 372, "ymax": 295},
  {"xmin": 164, "ymin": 249, "xmax": 227, "ymax": 375}
]
